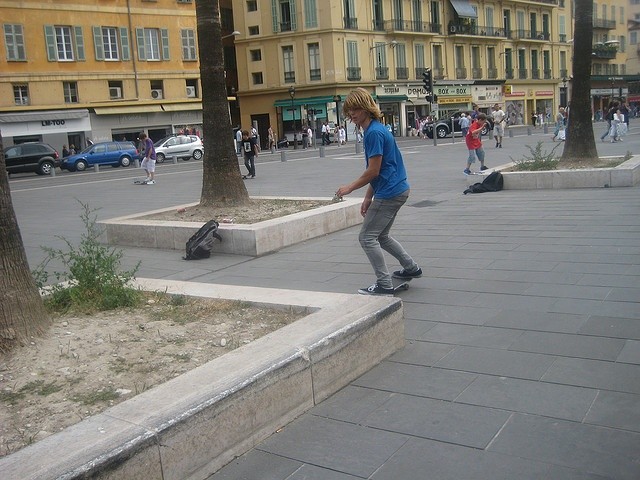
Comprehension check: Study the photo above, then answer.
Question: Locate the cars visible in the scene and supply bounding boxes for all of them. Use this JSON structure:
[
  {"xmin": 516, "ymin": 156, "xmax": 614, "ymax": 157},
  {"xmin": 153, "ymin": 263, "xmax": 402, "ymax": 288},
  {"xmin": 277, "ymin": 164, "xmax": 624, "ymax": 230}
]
[
  {"xmin": 140, "ymin": 134, "xmax": 204, "ymax": 163},
  {"xmin": 4, "ymin": 142, "xmax": 59, "ymax": 177},
  {"xmin": 422, "ymin": 110, "xmax": 491, "ymax": 138},
  {"xmin": 63, "ymin": 141, "xmax": 139, "ymax": 171}
]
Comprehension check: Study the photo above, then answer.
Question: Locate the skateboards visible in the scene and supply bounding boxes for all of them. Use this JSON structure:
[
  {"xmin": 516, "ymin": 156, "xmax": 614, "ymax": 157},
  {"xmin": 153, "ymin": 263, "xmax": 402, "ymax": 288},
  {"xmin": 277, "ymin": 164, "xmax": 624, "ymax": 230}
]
[
  {"xmin": 133, "ymin": 180, "xmax": 146, "ymax": 185},
  {"xmin": 242, "ymin": 175, "xmax": 252, "ymax": 179},
  {"xmin": 391, "ymin": 276, "xmax": 411, "ymax": 290}
]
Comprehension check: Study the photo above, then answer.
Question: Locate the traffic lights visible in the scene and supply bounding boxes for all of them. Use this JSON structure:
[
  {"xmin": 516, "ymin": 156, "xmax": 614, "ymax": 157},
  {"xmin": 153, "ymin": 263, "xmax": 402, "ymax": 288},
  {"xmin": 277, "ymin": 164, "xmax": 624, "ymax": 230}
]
[{"xmin": 422, "ymin": 72, "xmax": 432, "ymax": 93}]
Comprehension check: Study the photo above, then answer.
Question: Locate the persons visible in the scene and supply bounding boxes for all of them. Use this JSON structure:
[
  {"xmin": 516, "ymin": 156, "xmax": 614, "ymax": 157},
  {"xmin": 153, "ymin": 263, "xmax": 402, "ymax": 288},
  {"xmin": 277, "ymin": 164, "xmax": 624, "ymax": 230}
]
[
  {"xmin": 415, "ymin": 116, "xmax": 433, "ymax": 139},
  {"xmin": 470, "ymin": 105, "xmax": 481, "ymax": 120},
  {"xmin": 621, "ymin": 102, "xmax": 632, "ymax": 132},
  {"xmin": 331, "ymin": 86, "xmax": 422, "ymax": 298},
  {"xmin": 462, "ymin": 113, "xmax": 491, "ymax": 176},
  {"xmin": 86, "ymin": 137, "xmax": 94, "ymax": 146},
  {"xmin": 250, "ymin": 124, "xmax": 261, "ymax": 158},
  {"xmin": 69, "ymin": 144, "xmax": 76, "ymax": 155},
  {"xmin": 551, "ymin": 107, "xmax": 565, "ymax": 142},
  {"xmin": 308, "ymin": 126, "xmax": 312, "ymax": 147},
  {"xmin": 464, "ymin": 112, "xmax": 471, "ymax": 121},
  {"xmin": 321, "ymin": 122, "xmax": 330, "ymax": 145},
  {"xmin": 491, "ymin": 103, "xmax": 506, "ymax": 148},
  {"xmin": 385, "ymin": 121, "xmax": 391, "ymax": 131},
  {"xmin": 235, "ymin": 127, "xmax": 242, "ymax": 153},
  {"xmin": 457, "ymin": 114, "xmax": 467, "ymax": 138},
  {"xmin": 139, "ymin": 132, "xmax": 156, "ymax": 184},
  {"xmin": 268, "ymin": 125, "xmax": 274, "ymax": 149},
  {"xmin": 600, "ymin": 102, "xmax": 614, "ymax": 142},
  {"xmin": 325, "ymin": 122, "xmax": 331, "ymax": 145},
  {"xmin": 538, "ymin": 111, "xmax": 544, "ymax": 129},
  {"xmin": 334, "ymin": 123, "xmax": 338, "ymax": 145},
  {"xmin": 302, "ymin": 126, "xmax": 309, "ymax": 149},
  {"xmin": 353, "ymin": 124, "xmax": 362, "ymax": 142},
  {"xmin": 62, "ymin": 145, "xmax": 69, "ymax": 157},
  {"xmin": 610, "ymin": 102, "xmax": 623, "ymax": 142},
  {"xmin": 531, "ymin": 113, "xmax": 537, "ymax": 128},
  {"xmin": 341, "ymin": 126, "xmax": 346, "ymax": 144},
  {"xmin": 240, "ymin": 130, "xmax": 259, "ymax": 178}
]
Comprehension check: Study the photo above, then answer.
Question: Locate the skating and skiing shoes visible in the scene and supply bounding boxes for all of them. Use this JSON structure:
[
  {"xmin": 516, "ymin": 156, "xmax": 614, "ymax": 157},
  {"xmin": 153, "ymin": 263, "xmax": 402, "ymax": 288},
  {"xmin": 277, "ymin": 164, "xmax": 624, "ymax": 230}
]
[{"xmin": 496, "ymin": 143, "xmax": 502, "ymax": 148}]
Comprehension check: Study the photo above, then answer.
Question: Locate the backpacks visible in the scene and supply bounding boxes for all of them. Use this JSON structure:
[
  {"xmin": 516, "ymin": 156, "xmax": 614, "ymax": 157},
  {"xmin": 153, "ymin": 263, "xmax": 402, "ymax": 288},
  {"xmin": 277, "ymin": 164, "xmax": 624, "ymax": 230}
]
[
  {"xmin": 482, "ymin": 170, "xmax": 503, "ymax": 191},
  {"xmin": 463, "ymin": 183, "xmax": 486, "ymax": 195},
  {"xmin": 182, "ymin": 220, "xmax": 222, "ymax": 260}
]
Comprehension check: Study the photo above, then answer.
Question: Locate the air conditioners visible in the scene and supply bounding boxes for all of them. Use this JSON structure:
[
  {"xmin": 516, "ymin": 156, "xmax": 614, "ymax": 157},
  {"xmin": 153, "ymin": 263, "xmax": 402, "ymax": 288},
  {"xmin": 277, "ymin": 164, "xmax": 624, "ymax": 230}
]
[
  {"xmin": 150, "ymin": 89, "xmax": 162, "ymax": 100},
  {"xmin": 186, "ymin": 86, "xmax": 196, "ymax": 98},
  {"xmin": 109, "ymin": 86, "xmax": 121, "ymax": 99}
]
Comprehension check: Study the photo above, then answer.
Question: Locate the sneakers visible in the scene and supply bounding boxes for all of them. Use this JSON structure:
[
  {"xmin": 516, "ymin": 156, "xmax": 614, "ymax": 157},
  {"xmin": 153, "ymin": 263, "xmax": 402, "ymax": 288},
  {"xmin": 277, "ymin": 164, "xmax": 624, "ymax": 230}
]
[
  {"xmin": 357, "ymin": 284, "xmax": 394, "ymax": 296},
  {"xmin": 392, "ymin": 268, "xmax": 423, "ymax": 279},
  {"xmin": 463, "ymin": 168, "xmax": 471, "ymax": 174},
  {"xmin": 247, "ymin": 172, "xmax": 255, "ymax": 178},
  {"xmin": 480, "ymin": 166, "xmax": 489, "ymax": 171},
  {"xmin": 145, "ymin": 176, "xmax": 155, "ymax": 184}
]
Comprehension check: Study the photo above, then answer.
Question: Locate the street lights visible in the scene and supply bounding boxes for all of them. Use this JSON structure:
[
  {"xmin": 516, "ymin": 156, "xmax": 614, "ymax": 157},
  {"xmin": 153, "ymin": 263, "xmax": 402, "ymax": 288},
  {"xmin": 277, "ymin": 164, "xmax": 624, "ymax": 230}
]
[
  {"xmin": 370, "ymin": 41, "xmax": 397, "ymax": 49},
  {"xmin": 500, "ymin": 47, "xmax": 525, "ymax": 54},
  {"xmin": 221, "ymin": 31, "xmax": 241, "ymax": 39}
]
